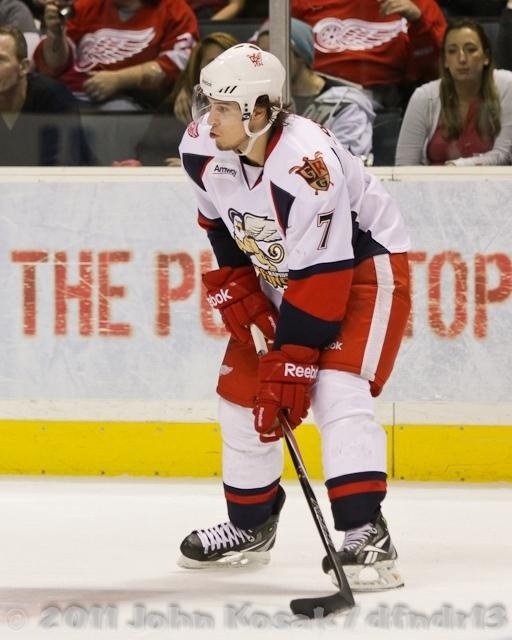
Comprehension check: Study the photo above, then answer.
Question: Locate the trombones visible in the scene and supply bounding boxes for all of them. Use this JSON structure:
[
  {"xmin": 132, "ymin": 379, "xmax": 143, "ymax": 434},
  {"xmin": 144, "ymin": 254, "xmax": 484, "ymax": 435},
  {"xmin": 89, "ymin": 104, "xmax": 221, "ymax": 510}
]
[{"xmin": 251, "ymin": 325, "xmax": 353, "ymax": 617}]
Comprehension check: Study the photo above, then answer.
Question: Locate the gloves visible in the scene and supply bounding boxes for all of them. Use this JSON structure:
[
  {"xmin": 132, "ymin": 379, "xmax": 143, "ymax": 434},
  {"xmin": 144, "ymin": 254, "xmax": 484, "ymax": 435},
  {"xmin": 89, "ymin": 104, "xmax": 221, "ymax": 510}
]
[
  {"xmin": 254, "ymin": 343, "xmax": 320, "ymax": 444},
  {"xmin": 200, "ymin": 264, "xmax": 278, "ymax": 347}
]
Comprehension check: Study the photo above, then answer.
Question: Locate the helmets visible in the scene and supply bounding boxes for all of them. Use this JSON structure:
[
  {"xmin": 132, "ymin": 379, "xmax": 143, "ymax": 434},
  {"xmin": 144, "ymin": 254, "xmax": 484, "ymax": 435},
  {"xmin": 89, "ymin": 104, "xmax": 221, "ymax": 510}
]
[{"xmin": 200, "ymin": 43, "xmax": 287, "ymax": 136}]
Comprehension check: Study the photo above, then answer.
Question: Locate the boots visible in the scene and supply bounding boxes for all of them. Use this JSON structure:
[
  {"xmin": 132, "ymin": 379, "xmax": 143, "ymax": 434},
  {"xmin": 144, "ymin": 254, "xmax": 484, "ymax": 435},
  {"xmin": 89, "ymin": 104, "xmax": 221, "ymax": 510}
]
[
  {"xmin": 323, "ymin": 510, "xmax": 398, "ymax": 572},
  {"xmin": 179, "ymin": 485, "xmax": 286, "ymax": 561}
]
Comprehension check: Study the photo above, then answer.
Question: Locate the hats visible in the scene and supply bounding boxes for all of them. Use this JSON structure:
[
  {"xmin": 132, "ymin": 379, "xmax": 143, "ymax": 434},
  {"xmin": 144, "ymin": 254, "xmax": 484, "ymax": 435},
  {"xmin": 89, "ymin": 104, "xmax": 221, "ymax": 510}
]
[{"xmin": 257, "ymin": 17, "xmax": 315, "ymax": 67}]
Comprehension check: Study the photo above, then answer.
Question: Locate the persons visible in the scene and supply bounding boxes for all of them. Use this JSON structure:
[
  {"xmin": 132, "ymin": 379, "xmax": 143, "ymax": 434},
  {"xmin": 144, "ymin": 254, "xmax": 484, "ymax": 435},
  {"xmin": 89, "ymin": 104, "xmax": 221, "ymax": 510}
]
[
  {"xmin": 0, "ymin": 1, "xmax": 511, "ymax": 165},
  {"xmin": 178, "ymin": 43, "xmax": 411, "ymax": 591}
]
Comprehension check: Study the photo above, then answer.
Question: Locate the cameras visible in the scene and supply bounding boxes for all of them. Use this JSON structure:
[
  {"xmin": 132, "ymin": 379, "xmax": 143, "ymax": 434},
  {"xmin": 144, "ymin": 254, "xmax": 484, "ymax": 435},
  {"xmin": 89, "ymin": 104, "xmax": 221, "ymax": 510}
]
[{"xmin": 57, "ymin": 4, "xmax": 75, "ymax": 19}]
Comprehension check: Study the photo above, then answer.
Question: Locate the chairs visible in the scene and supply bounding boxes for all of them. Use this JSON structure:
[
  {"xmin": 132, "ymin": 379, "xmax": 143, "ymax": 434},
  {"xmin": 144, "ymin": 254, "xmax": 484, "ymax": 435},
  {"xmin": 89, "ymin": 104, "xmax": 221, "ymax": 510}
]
[{"xmin": 0, "ymin": 1, "xmax": 511, "ymax": 165}]
